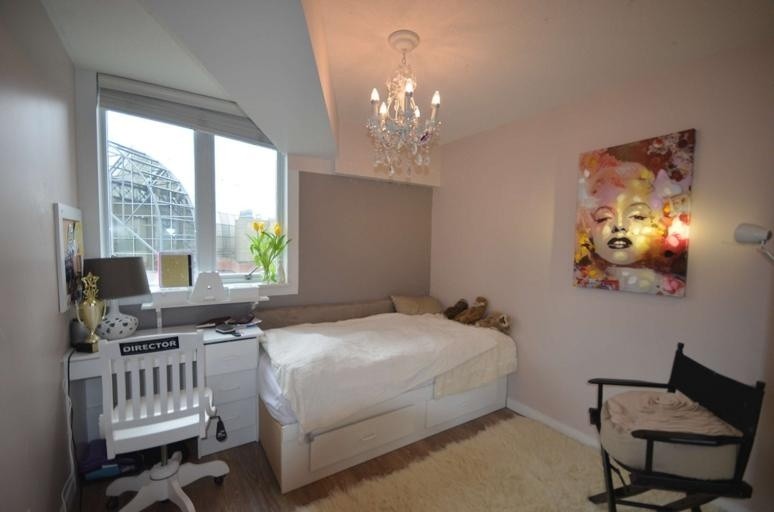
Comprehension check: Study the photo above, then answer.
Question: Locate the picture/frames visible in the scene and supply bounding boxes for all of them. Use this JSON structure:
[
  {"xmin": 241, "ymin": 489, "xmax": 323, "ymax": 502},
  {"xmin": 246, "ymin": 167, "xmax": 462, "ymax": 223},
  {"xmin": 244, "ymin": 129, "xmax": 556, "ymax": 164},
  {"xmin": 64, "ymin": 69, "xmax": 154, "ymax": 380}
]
[
  {"xmin": 158, "ymin": 250, "xmax": 194, "ymax": 289},
  {"xmin": 54, "ymin": 202, "xmax": 85, "ymax": 314}
]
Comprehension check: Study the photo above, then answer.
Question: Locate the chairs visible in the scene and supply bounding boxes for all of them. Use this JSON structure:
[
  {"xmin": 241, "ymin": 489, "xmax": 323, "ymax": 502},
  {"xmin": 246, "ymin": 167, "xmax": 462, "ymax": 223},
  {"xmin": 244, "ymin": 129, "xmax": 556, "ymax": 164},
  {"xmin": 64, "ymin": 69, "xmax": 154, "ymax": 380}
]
[
  {"xmin": 97, "ymin": 330, "xmax": 231, "ymax": 512},
  {"xmin": 584, "ymin": 341, "xmax": 766, "ymax": 512}
]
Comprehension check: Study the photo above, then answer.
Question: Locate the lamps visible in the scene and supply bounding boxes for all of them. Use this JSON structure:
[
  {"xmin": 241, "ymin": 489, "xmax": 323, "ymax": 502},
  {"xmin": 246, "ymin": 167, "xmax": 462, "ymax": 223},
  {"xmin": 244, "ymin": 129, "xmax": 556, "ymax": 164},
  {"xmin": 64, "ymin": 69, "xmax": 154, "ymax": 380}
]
[
  {"xmin": 733, "ymin": 222, "xmax": 774, "ymax": 266},
  {"xmin": 364, "ymin": 29, "xmax": 443, "ymax": 180},
  {"xmin": 84, "ymin": 256, "xmax": 152, "ymax": 341}
]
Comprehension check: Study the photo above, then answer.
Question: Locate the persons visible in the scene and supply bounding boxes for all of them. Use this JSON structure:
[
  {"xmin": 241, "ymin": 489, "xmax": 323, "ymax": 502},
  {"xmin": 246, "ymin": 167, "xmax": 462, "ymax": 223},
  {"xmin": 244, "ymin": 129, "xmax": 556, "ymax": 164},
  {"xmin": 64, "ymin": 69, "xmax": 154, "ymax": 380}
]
[
  {"xmin": 575, "ymin": 161, "xmax": 685, "ymax": 298},
  {"xmin": 64, "ymin": 223, "xmax": 79, "ymax": 294}
]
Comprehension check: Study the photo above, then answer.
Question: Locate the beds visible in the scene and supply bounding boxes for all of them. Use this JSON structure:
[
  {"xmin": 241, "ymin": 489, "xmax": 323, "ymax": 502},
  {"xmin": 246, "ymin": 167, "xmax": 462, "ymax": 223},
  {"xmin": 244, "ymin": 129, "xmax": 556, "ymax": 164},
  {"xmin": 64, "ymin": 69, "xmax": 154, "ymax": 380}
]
[{"xmin": 247, "ymin": 296, "xmax": 519, "ymax": 497}]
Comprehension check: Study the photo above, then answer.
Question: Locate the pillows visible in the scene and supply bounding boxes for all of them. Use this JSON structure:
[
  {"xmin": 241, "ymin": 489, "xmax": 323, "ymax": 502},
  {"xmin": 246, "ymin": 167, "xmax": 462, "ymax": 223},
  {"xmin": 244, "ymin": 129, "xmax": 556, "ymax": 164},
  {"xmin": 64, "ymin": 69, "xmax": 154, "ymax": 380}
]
[
  {"xmin": 390, "ymin": 295, "xmax": 446, "ymax": 318},
  {"xmin": 600, "ymin": 391, "xmax": 737, "ymax": 480}
]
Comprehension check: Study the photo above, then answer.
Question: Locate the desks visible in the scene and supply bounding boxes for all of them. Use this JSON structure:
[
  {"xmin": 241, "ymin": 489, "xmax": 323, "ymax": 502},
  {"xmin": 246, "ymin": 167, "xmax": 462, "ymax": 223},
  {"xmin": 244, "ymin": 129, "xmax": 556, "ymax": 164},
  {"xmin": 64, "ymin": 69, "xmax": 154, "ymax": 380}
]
[{"xmin": 62, "ymin": 317, "xmax": 265, "ymax": 460}]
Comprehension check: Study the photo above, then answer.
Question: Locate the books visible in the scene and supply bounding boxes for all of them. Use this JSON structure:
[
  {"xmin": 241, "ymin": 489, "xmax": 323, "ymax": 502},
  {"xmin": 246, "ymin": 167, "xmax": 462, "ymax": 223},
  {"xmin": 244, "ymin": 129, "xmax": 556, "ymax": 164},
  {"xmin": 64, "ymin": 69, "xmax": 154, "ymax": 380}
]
[
  {"xmin": 196, "ymin": 316, "xmax": 232, "ymax": 328},
  {"xmin": 225, "ymin": 314, "xmax": 255, "ymax": 324},
  {"xmin": 234, "ymin": 318, "xmax": 263, "ymax": 328},
  {"xmin": 216, "ymin": 324, "xmax": 236, "ymax": 334}
]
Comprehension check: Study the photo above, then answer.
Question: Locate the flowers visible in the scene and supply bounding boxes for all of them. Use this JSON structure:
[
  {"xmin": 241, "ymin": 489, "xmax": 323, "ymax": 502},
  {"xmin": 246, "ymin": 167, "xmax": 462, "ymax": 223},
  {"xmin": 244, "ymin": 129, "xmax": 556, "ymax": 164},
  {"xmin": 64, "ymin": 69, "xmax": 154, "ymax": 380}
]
[{"xmin": 243, "ymin": 220, "xmax": 291, "ymax": 282}]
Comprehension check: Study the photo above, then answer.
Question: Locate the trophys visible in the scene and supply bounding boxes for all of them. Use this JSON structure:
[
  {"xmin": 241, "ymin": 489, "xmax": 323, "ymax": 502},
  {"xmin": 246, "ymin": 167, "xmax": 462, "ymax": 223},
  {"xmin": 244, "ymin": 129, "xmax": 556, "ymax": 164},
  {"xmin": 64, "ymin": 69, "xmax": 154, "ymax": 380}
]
[{"xmin": 71, "ymin": 271, "xmax": 108, "ymax": 355}]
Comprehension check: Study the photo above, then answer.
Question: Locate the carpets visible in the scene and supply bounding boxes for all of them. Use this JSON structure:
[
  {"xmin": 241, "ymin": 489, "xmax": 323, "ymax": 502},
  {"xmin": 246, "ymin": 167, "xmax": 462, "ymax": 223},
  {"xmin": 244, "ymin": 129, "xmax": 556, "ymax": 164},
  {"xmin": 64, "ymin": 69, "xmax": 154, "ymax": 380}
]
[{"xmin": 295, "ymin": 414, "xmax": 760, "ymax": 512}]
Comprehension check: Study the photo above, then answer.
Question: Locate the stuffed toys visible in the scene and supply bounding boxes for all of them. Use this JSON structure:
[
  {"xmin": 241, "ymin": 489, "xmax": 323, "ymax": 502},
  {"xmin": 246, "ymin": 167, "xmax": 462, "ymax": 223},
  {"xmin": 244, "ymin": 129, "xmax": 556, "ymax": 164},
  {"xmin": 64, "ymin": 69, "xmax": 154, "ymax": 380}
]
[
  {"xmin": 454, "ymin": 296, "xmax": 488, "ymax": 325},
  {"xmin": 444, "ymin": 298, "xmax": 470, "ymax": 321},
  {"xmin": 475, "ymin": 310, "xmax": 512, "ymax": 336}
]
